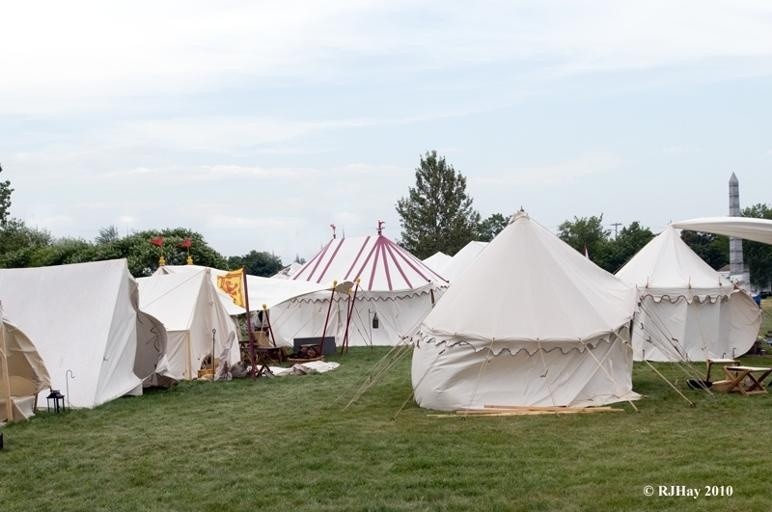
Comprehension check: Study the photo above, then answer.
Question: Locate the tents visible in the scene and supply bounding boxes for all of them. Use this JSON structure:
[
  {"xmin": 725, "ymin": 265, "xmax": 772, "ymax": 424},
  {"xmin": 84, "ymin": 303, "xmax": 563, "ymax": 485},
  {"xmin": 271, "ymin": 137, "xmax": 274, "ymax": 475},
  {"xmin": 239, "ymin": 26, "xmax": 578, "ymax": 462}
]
[
  {"xmin": 613, "ymin": 221, "xmax": 764, "ymax": 362},
  {"xmin": 409, "ymin": 205, "xmax": 643, "ymax": 412}
]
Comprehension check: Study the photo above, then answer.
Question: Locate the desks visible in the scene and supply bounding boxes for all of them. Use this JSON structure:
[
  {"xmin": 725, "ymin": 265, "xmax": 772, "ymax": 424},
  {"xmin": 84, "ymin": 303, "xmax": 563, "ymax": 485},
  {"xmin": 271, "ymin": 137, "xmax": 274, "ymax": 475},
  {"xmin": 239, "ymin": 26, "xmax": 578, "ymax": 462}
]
[
  {"xmin": 241, "ymin": 347, "xmax": 281, "ymax": 378},
  {"xmin": 722, "ymin": 365, "xmax": 772, "ymax": 395}
]
